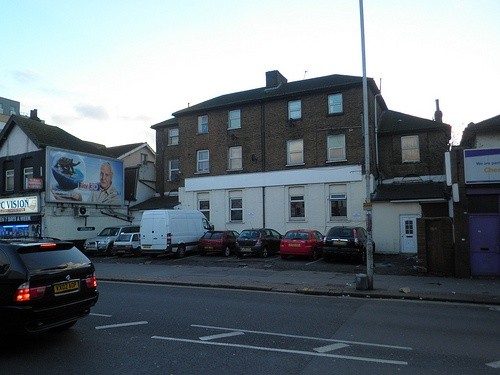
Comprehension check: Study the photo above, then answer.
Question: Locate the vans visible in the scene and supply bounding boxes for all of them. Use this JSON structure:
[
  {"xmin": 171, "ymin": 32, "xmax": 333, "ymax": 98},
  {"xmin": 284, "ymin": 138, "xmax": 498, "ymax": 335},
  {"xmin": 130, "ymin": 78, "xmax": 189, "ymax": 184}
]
[{"xmin": 140, "ymin": 210, "xmax": 214, "ymax": 256}]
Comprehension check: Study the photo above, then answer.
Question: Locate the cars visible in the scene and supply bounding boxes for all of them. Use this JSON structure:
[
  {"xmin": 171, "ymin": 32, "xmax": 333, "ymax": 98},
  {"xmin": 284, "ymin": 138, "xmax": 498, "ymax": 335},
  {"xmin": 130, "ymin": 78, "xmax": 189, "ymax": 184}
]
[
  {"xmin": 200, "ymin": 230, "xmax": 240, "ymax": 256},
  {"xmin": 280, "ymin": 229, "xmax": 323, "ymax": 257},
  {"xmin": 114, "ymin": 233, "xmax": 141, "ymax": 255},
  {"xmin": 321, "ymin": 225, "xmax": 375, "ymax": 262},
  {"xmin": 237, "ymin": 228, "xmax": 283, "ymax": 257}
]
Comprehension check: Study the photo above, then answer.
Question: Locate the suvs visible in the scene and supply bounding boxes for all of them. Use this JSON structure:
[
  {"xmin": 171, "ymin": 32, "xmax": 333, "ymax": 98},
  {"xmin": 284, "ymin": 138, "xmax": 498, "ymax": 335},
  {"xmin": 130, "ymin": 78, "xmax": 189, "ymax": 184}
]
[
  {"xmin": 84, "ymin": 225, "xmax": 141, "ymax": 255},
  {"xmin": 0, "ymin": 236, "xmax": 100, "ymax": 346}
]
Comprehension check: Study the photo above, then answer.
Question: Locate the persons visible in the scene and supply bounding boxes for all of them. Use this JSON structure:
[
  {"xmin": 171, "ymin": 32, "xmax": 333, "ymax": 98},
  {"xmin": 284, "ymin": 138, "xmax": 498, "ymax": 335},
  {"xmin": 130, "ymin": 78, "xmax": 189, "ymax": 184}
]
[{"xmin": 88, "ymin": 161, "xmax": 121, "ymax": 203}]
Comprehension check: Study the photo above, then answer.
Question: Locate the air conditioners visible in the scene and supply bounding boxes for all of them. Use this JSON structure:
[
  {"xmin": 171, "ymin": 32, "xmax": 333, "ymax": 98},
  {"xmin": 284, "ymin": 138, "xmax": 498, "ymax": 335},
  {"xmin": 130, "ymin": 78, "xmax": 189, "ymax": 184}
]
[{"xmin": 74, "ymin": 206, "xmax": 91, "ymax": 216}]
